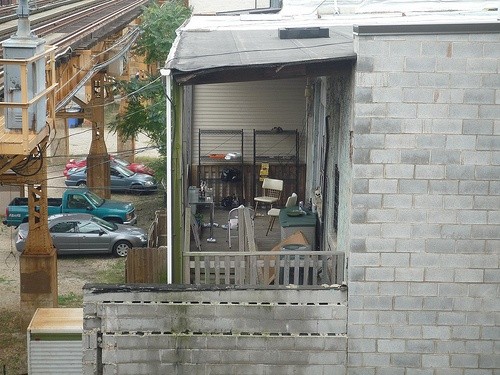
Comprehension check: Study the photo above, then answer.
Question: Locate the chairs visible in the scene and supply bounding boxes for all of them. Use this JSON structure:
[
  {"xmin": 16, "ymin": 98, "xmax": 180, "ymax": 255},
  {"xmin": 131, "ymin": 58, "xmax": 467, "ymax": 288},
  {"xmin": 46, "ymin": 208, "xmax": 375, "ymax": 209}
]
[
  {"xmin": 226, "ymin": 207, "xmax": 256, "ymax": 249},
  {"xmin": 253, "ymin": 178, "xmax": 283, "ymax": 216},
  {"xmin": 265, "ymin": 192, "xmax": 297, "ymax": 236}
]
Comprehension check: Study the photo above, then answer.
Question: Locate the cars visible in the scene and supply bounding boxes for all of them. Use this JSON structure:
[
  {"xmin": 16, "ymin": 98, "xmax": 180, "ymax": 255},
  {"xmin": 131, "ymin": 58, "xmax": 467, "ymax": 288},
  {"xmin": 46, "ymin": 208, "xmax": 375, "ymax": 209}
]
[
  {"xmin": 63, "ymin": 154, "xmax": 157, "ymax": 195},
  {"xmin": 15, "ymin": 213, "xmax": 148, "ymax": 258}
]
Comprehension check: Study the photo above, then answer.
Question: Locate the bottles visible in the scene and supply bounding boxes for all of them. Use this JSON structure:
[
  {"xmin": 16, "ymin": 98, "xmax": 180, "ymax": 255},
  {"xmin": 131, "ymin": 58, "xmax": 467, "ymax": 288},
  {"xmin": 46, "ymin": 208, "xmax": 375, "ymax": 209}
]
[
  {"xmin": 202, "ymin": 190, "xmax": 205, "ymax": 199},
  {"xmin": 309, "ymin": 201, "xmax": 312, "ymax": 209},
  {"xmin": 199, "ymin": 190, "xmax": 201, "ymax": 199}
]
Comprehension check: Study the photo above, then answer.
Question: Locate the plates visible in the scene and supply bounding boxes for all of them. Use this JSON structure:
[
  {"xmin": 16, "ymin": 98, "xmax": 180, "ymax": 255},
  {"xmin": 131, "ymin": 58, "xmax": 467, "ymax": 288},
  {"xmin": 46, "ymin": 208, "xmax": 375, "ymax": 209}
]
[{"xmin": 287, "ymin": 210, "xmax": 304, "ymax": 216}]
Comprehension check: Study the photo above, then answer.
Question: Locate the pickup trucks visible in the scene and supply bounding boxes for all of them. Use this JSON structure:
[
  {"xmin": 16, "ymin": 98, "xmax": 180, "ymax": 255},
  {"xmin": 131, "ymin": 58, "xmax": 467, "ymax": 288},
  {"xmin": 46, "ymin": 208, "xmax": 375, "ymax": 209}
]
[{"xmin": 3, "ymin": 187, "xmax": 138, "ymax": 227}]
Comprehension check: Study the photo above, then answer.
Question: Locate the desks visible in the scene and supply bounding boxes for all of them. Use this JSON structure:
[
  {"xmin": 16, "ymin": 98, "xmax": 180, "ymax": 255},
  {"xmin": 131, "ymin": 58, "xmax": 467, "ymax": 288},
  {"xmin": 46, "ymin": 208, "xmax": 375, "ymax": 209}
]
[
  {"xmin": 188, "ymin": 198, "xmax": 217, "ymax": 243},
  {"xmin": 279, "ymin": 208, "xmax": 316, "ymax": 251}
]
